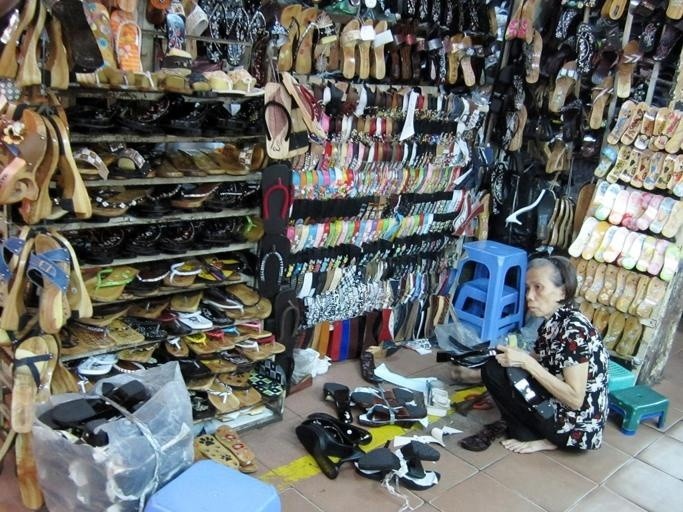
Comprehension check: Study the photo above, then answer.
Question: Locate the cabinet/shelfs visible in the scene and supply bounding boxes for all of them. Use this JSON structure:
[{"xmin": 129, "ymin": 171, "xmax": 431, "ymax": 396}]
[{"xmin": 21, "ymin": 70, "xmax": 291, "ymax": 436}]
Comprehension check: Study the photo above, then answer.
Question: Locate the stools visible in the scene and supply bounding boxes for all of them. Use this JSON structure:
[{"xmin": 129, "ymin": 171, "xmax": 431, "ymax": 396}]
[
  {"xmin": 603, "ymin": 355, "xmax": 636, "ymax": 390},
  {"xmin": 605, "ymin": 384, "xmax": 670, "ymax": 434},
  {"xmin": 446, "ymin": 239, "xmax": 527, "ymax": 346},
  {"xmin": 448, "ymin": 277, "xmax": 519, "ymax": 355},
  {"xmin": 143, "ymin": 454, "xmax": 284, "ymax": 511}
]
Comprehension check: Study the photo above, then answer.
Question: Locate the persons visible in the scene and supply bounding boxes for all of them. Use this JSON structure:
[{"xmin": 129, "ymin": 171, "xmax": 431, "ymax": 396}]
[{"xmin": 447, "ymin": 255, "xmax": 609, "ymax": 454}]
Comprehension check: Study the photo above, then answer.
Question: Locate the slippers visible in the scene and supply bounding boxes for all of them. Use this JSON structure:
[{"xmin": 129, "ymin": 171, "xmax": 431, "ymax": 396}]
[
  {"xmin": 1, "ymin": 2, "xmax": 299, "ymax": 509},
  {"xmin": 301, "ymin": 2, "xmax": 682, "ymax": 384}
]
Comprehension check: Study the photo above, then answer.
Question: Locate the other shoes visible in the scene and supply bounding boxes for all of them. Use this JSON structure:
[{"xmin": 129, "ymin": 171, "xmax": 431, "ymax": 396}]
[
  {"xmin": 462, "ymin": 421, "xmax": 507, "ymax": 451},
  {"xmin": 295, "ymin": 382, "xmax": 440, "ymax": 491},
  {"xmin": 457, "ymin": 390, "xmax": 491, "ymax": 416}
]
